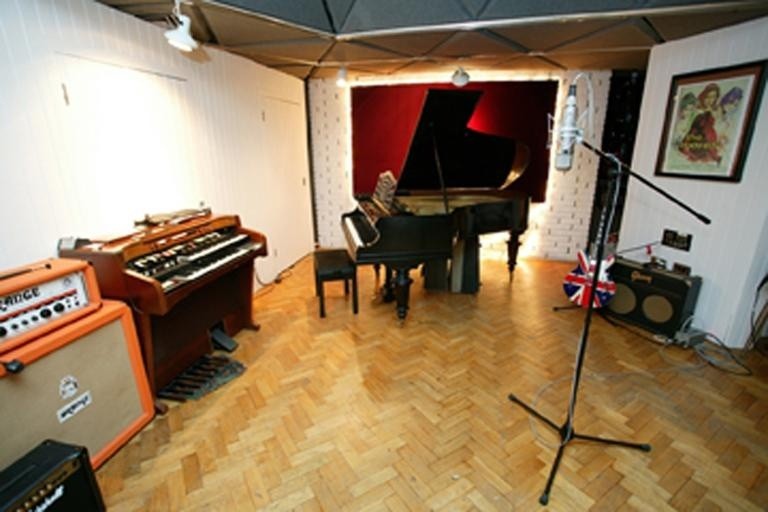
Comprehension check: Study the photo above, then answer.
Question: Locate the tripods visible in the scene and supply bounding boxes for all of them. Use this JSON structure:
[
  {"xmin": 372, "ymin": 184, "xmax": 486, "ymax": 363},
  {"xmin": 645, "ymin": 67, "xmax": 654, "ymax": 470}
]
[{"xmin": 508, "ymin": 137, "xmax": 711, "ymax": 506}]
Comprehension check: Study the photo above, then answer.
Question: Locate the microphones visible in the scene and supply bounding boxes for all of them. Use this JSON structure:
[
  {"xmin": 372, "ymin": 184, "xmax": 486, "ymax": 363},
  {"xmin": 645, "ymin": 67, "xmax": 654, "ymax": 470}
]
[{"xmin": 555, "ymin": 84, "xmax": 576, "ymax": 171}]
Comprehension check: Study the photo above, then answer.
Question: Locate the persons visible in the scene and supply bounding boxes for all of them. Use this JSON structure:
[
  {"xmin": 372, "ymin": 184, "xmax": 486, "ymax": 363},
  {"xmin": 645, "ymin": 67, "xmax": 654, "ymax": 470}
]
[
  {"xmin": 674, "ymin": 91, "xmax": 699, "ymax": 145},
  {"xmin": 716, "ymin": 86, "xmax": 745, "ymax": 148},
  {"xmin": 678, "ymin": 78, "xmax": 726, "ymax": 167}
]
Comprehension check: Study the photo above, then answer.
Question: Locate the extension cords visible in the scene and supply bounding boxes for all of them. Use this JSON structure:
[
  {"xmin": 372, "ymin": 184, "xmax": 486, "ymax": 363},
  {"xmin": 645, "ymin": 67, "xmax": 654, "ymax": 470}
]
[{"xmin": 673, "ymin": 328, "xmax": 705, "ymax": 349}]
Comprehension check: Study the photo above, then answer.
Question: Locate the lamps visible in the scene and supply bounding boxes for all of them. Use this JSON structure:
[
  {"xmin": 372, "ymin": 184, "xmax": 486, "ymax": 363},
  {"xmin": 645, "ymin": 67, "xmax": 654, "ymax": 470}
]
[
  {"xmin": 451, "ymin": 68, "xmax": 471, "ymax": 88},
  {"xmin": 164, "ymin": 0, "xmax": 199, "ymax": 53},
  {"xmin": 335, "ymin": 64, "xmax": 348, "ymax": 88}
]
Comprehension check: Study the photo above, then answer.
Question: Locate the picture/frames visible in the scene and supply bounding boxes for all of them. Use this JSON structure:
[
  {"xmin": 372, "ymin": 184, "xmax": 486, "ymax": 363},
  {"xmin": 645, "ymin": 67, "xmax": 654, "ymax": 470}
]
[{"xmin": 654, "ymin": 58, "xmax": 768, "ymax": 182}]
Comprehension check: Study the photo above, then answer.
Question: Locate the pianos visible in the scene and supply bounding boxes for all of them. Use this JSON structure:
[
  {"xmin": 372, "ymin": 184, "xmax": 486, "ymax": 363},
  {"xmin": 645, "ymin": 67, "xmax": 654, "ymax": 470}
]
[
  {"xmin": 60, "ymin": 208, "xmax": 269, "ymax": 401},
  {"xmin": 341, "ymin": 88, "xmax": 530, "ymax": 325}
]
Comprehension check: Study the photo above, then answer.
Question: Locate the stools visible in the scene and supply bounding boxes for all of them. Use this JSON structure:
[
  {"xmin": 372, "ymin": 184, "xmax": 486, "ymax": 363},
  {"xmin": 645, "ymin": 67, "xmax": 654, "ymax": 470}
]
[{"xmin": 313, "ymin": 249, "xmax": 359, "ymax": 317}]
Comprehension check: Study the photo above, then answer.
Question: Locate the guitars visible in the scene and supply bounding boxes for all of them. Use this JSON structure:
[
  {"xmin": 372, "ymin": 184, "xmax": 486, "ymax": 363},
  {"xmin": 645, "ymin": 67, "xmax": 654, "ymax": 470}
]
[{"xmin": 564, "ymin": 170, "xmax": 619, "ymax": 309}]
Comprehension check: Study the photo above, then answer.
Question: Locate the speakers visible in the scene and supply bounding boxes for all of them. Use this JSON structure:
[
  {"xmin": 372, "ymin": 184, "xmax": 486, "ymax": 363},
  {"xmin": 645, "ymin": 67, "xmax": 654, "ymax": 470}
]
[
  {"xmin": 0, "ymin": 299, "xmax": 155, "ymax": 471},
  {"xmin": 604, "ymin": 255, "xmax": 701, "ymax": 336},
  {"xmin": 0, "ymin": 439, "xmax": 106, "ymax": 512}
]
